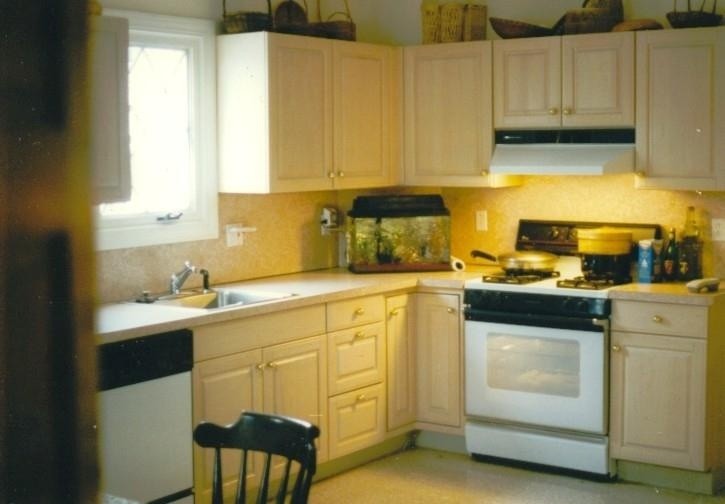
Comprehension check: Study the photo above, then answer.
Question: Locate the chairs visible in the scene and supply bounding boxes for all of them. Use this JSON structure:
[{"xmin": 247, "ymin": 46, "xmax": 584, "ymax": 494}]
[{"xmin": 193, "ymin": 410, "xmax": 321, "ymax": 504}]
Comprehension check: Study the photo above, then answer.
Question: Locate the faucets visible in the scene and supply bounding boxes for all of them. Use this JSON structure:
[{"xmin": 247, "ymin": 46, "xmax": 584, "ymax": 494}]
[{"xmin": 169, "ymin": 260, "xmax": 198, "ymax": 294}]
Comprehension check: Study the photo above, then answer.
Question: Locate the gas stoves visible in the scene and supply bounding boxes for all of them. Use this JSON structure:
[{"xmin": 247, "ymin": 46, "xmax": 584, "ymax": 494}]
[{"xmin": 464, "ymin": 219, "xmax": 659, "ymax": 299}]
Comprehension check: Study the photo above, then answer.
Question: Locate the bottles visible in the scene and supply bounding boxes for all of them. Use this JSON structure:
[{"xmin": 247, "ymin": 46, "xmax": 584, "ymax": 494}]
[
  {"xmin": 678, "ymin": 207, "xmax": 700, "ymax": 282},
  {"xmin": 662, "ymin": 227, "xmax": 677, "ymax": 281}
]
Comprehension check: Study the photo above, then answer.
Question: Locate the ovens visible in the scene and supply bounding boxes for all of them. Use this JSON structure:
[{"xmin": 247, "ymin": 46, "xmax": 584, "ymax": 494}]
[{"xmin": 462, "ymin": 290, "xmax": 618, "ymax": 479}]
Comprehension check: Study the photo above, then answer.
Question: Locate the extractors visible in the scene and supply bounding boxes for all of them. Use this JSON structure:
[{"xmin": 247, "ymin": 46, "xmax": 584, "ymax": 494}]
[{"xmin": 488, "ymin": 130, "xmax": 635, "ymax": 177}]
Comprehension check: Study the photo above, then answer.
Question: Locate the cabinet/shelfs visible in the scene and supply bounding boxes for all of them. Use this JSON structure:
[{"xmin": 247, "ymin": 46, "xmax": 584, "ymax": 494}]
[
  {"xmin": 326, "ymin": 288, "xmax": 462, "ymax": 480},
  {"xmin": 192, "ymin": 303, "xmax": 327, "ymax": 504},
  {"xmin": 608, "ymin": 282, "xmax": 725, "ymax": 496},
  {"xmin": 88, "ymin": 16, "xmax": 133, "ymax": 207}
]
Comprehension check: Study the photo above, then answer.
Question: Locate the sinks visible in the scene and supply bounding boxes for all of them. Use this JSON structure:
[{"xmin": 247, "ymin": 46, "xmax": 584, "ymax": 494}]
[{"xmin": 125, "ymin": 285, "xmax": 302, "ymax": 311}]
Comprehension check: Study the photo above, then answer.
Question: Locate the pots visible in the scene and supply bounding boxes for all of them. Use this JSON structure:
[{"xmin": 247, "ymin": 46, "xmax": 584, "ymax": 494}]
[
  {"xmin": 471, "ymin": 250, "xmax": 560, "ymax": 274},
  {"xmin": 576, "ymin": 225, "xmax": 634, "ymax": 281}
]
